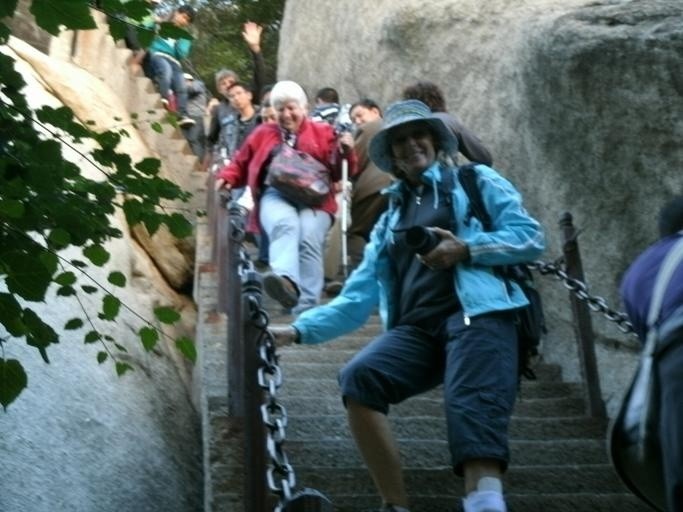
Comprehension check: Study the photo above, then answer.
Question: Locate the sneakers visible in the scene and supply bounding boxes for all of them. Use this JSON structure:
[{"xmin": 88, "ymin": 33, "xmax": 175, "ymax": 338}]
[
  {"xmin": 255, "ymin": 258, "xmax": 269, "ymax": 268},
  {"xmin": 463, "ymin": 477, "xmax": 506, "ymax": 511},
  {"xmin": 265, "ymin": 274, "xmax": 298, "ymax": 308},
  {"xmin": 176, "ymin": 115, "xmax": 196, "ymax": 128},
  {"xmin": 325, "ymin": 277, "xmax": 343, "ymax": 292}
]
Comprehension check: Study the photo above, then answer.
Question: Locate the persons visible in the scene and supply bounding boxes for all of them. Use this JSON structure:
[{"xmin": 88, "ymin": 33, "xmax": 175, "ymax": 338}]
[
  {"xmin": 262, "ymin": 98, "xmax": 545, "ymax": 512},
  {"xmin": 399, "ymin": 80, "xmax": 494, "ymax": 169},
  {"xmin": 128, "ymin": 5, "xmax": 361, "ymax": 316},
  {"xmin": 347, "ymin": 96, "xmax": 394, "ymax": 275},
  {"xmin": 617, "ymin": 192, "xmax": 682, "ymax": 512}
]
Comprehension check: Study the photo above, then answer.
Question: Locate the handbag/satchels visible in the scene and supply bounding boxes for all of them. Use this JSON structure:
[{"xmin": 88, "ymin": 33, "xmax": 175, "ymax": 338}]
[
  {"xmin": 268, "ymin": 143, "xmax": 330, "ymax": 202},
  {"xmin": 506, "ymin": 263, "xmax": 547, "ymax": 382},
  {"xmin": 610, "ymin": 357, "xmax": 668, "ymax": 511},
  {"xmin": 203, "ymin": 98, "xmax": 220, "ymax": 136}
]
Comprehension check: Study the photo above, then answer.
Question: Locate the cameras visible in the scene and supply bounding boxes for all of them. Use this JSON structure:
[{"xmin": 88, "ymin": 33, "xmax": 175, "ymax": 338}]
[{"xmin": 405, "ymin": 223, "xmax": 437, "ymax": 254}]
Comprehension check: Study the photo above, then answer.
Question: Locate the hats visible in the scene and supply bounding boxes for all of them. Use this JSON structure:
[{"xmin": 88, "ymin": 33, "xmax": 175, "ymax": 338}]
[{"xmin": 368, "ymin": 100, "xmax": 458, "ymax": 171}]
